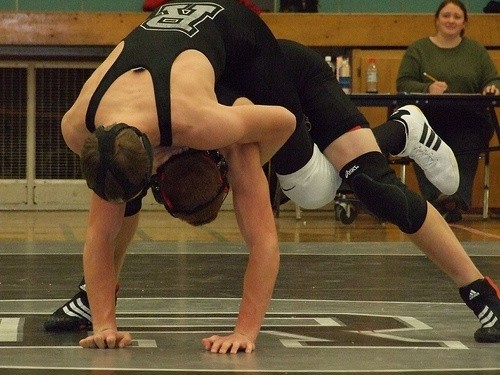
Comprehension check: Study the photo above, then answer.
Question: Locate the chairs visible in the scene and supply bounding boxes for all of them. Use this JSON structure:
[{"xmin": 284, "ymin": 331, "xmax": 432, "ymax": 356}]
[{"xmin": 384, "ymin": 103, "xmax": 491, "ymax": 219}]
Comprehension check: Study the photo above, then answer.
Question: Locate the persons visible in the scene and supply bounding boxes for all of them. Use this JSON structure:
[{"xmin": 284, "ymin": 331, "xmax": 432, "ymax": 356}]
[
  {"xmin": 44, "ymin": 39, "xmax": 500, "ymax": 342},
  {"xmin": 61, "ymin": 0, "xmax": 460, "ymax": 354},
  {"xmin": 396, "ymin": 0, "xmax": 500, "ymax": 224}
]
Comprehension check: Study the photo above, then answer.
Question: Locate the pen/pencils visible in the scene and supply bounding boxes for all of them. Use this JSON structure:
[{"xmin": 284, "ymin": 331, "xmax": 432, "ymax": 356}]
[{"xmin": 423, "ymin": 73, "xmax": 438, "ymax": 82}]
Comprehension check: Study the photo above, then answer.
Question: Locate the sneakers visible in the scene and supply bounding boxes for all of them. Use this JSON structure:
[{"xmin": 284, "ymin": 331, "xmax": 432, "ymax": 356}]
[
  {"xmin": 44, "ymin": 276, "xmax": 121, "ymax": 331},
  {"xmin": 458, "ymin": 276, "xmax": 500, "ymax": 342},
  {"xmin": 390, "ymin": 105, "xmax": 460, "ymax": 195}
]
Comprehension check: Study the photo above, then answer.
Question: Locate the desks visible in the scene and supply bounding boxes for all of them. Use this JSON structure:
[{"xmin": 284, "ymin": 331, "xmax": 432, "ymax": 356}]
[{"xmin": 344, "ymin": 89, "xmax": 500, "ymax": 220}]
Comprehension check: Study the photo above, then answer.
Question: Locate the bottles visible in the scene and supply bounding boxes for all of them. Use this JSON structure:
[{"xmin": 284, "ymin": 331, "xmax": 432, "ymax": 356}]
[
  {"xmin": 324, "ymin": 56, "xmax": 335, "ymax": 73},
  {"xmin": 365, "ymin": 57, "xmax": 378, "ymax": 93},
  {"xmin": 338, "ymin": 56, "xmax": 352, "ymax": 95}
]
